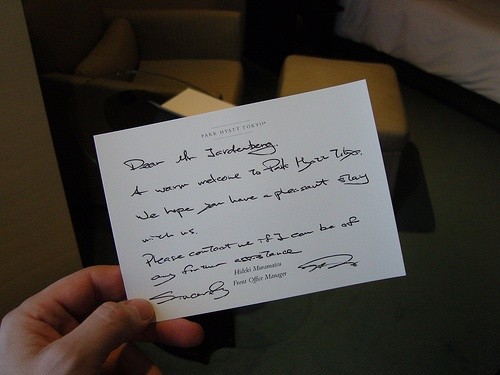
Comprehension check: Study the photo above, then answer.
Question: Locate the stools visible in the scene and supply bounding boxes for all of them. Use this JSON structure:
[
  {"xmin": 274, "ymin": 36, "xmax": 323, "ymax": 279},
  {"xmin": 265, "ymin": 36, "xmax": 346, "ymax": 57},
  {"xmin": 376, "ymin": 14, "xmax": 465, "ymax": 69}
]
[{"xmin": 276, "ymin": 56, "xmax": 408, "ymax": 200}]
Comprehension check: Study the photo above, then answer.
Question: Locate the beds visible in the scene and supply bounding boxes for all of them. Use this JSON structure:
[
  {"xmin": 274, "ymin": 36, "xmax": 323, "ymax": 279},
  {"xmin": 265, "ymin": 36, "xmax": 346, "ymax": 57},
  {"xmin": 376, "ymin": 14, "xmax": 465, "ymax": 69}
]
[{"xmin": 333, "ymin": 0, "xmax": 500, "ymax": 129}]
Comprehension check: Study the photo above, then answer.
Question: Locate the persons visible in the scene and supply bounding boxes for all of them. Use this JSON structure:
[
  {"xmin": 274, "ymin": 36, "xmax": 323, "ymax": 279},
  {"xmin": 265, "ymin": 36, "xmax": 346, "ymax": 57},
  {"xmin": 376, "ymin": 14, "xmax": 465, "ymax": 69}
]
[{"xmin": 0, "ymin": 264, "xmax": 205, "ymax": 375}]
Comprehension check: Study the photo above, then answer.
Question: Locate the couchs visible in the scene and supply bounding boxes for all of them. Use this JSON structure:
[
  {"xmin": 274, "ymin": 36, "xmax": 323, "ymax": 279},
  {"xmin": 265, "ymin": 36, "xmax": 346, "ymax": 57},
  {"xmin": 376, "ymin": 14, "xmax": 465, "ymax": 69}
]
[{"xmin": 20, "ymin": 1, "xmax": 242, "ymax": 142}]
